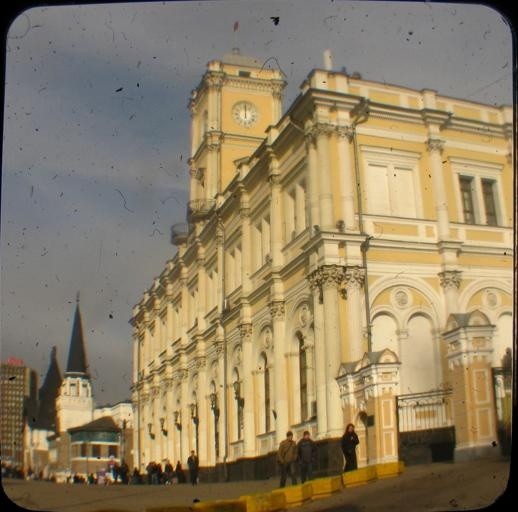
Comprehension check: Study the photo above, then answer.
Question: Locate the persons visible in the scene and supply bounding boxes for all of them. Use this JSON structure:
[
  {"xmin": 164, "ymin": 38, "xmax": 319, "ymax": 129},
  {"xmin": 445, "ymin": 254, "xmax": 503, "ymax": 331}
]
[
  {"xmin": 73, "ymin": 450, "xmax": 199, "ymax": 487},
  {"xmin": 340, "ymin": 423, "xmax": 358, "ymax": 473},
  {"xmin": 276, "ymin": 430, "xmax": 317, "ymax": 487}
]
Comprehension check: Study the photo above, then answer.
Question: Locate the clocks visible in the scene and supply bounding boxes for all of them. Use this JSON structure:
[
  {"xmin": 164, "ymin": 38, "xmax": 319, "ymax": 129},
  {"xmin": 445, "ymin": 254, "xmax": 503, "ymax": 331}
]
[{"xmin": 232, "ymin": 101, "xmax": 257, "ymax": 127}]
